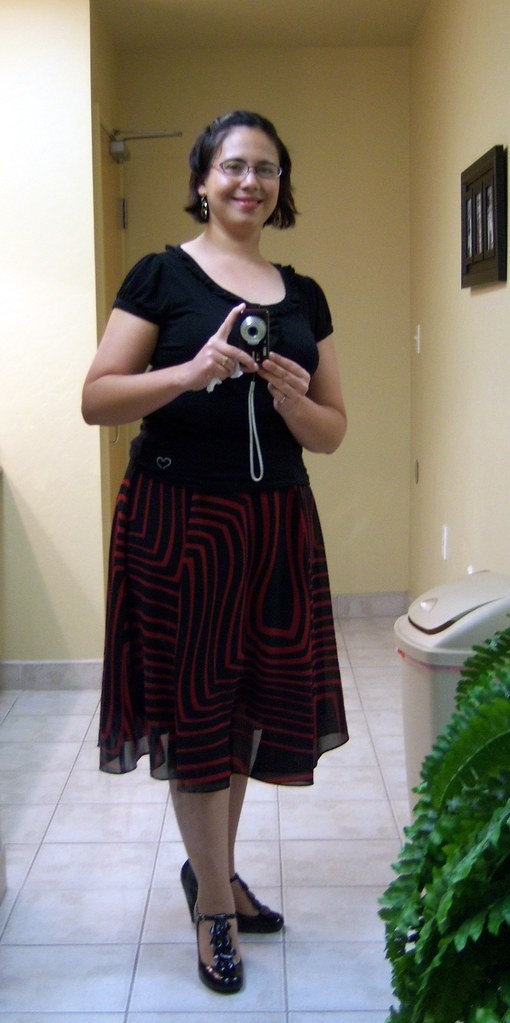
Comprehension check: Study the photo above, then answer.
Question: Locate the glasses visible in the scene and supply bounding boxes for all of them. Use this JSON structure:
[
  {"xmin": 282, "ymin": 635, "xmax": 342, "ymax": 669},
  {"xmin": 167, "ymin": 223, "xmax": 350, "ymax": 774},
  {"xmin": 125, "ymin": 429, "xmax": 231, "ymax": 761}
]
[{"xmin": 209, "ymin": 160, "xmax": 283, "ymax": 179}]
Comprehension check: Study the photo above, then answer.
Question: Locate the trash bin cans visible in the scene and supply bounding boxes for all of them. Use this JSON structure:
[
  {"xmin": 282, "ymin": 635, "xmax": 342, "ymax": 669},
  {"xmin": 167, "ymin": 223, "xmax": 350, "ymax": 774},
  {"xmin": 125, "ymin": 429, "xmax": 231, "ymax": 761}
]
[{"xmin": 394, "ymin": 569, "xmax": 510, "ymax": 818}]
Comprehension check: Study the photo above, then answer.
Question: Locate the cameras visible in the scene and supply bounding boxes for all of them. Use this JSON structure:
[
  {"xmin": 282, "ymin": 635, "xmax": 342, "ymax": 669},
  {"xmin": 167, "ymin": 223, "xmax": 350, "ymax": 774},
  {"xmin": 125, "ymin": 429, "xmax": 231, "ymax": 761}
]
[{"xmin": 238, "ymin": 310, "xmax": 270, "ymax": 364}]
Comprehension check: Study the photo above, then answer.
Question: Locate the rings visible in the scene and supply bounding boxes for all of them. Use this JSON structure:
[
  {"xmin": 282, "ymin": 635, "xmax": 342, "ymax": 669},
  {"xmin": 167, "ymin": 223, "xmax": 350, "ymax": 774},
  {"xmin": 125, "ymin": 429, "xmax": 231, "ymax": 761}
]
[
  {"xmin": 280, "ymin": 396, "xmax": 286, "ymax": 404},
  {"xmin": 222, "ymin": 356, "xmax": 229, "ymax": 368}
]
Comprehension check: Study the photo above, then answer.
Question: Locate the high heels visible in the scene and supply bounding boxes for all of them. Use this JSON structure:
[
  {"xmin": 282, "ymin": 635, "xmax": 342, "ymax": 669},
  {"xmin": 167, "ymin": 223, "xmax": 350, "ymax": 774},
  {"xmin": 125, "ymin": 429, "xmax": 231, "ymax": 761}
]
[
  {"xmin": 194, "ymin": 900, "xmax": 243, "ymax": 992},
  {"xmin": 180, "ymin": 858, "xmax": 284, "ymax": 934}
]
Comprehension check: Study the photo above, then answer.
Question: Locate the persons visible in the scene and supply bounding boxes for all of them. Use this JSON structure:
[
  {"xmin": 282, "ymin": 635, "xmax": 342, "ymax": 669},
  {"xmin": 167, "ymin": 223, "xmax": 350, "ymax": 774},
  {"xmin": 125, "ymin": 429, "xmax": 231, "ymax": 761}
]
[{"xmin": 81, "ymin": 110, "xmax": 347, "ymax": 995}]
